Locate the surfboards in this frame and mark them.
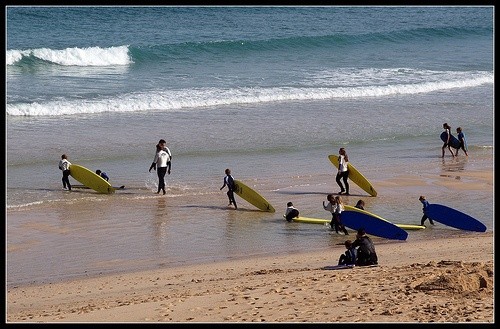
[340,204,408,241]
[68,183,126,190]
[328,155,378,197]
[284,214,425,230]
[423,203,486,233]
[232,180,275,212]
[439,130,462,149]
[68,164,115,194]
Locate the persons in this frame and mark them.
[59,155,72,190]
[336,148,350,195]
[353,200,364,210]
[149,139,172,195]
[286,202,299,223]
[351,228,375,265]
[455,127,468,156]
[338,240,359,267]
[220,169,237,210]
[441,123,455,157]
[323,194,350,236]
[96,170,109,181]
[419,196,434,226]
